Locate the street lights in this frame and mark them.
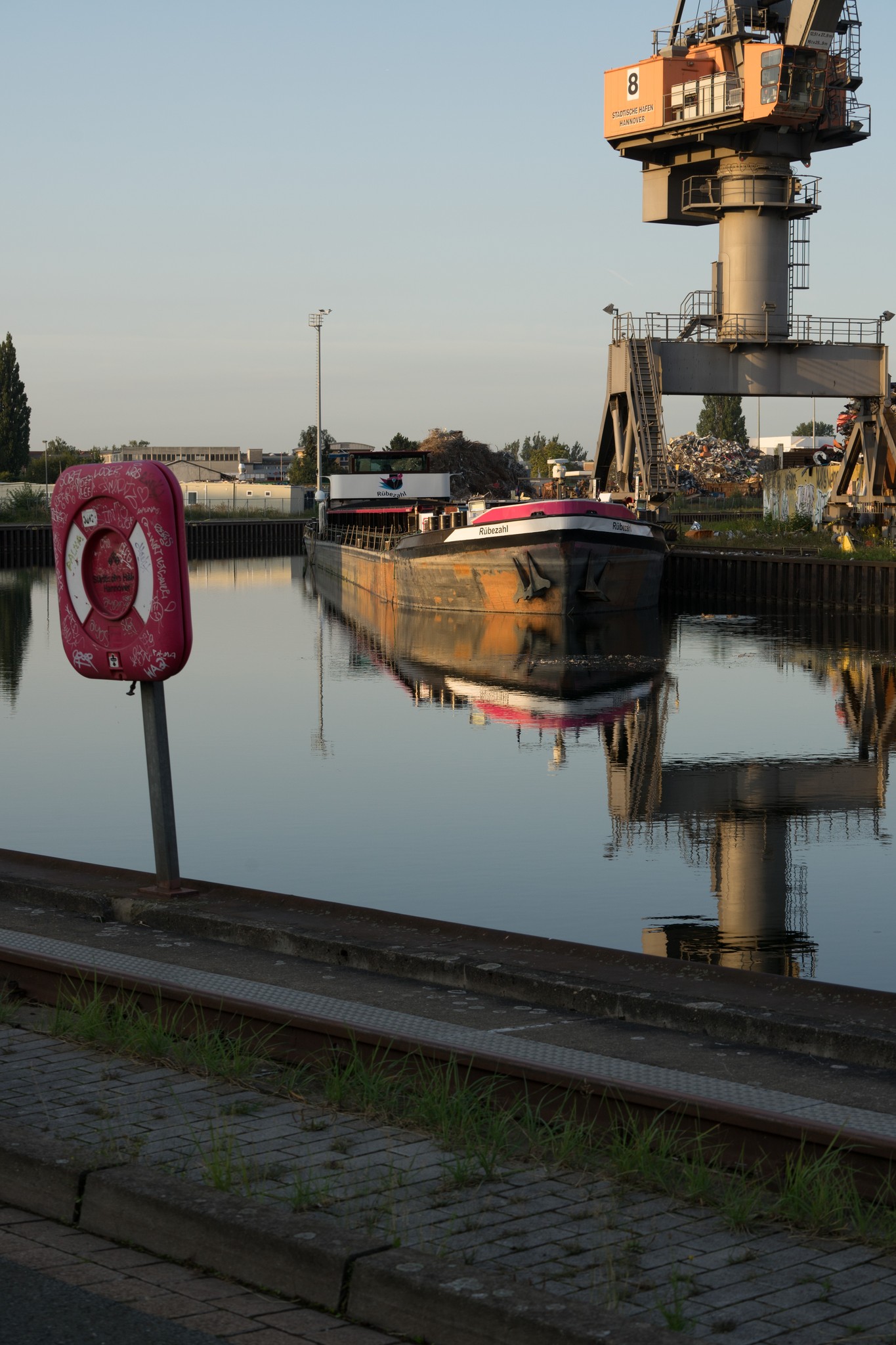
[42,441,48,509]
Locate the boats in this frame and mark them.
[303,451,675,689]
